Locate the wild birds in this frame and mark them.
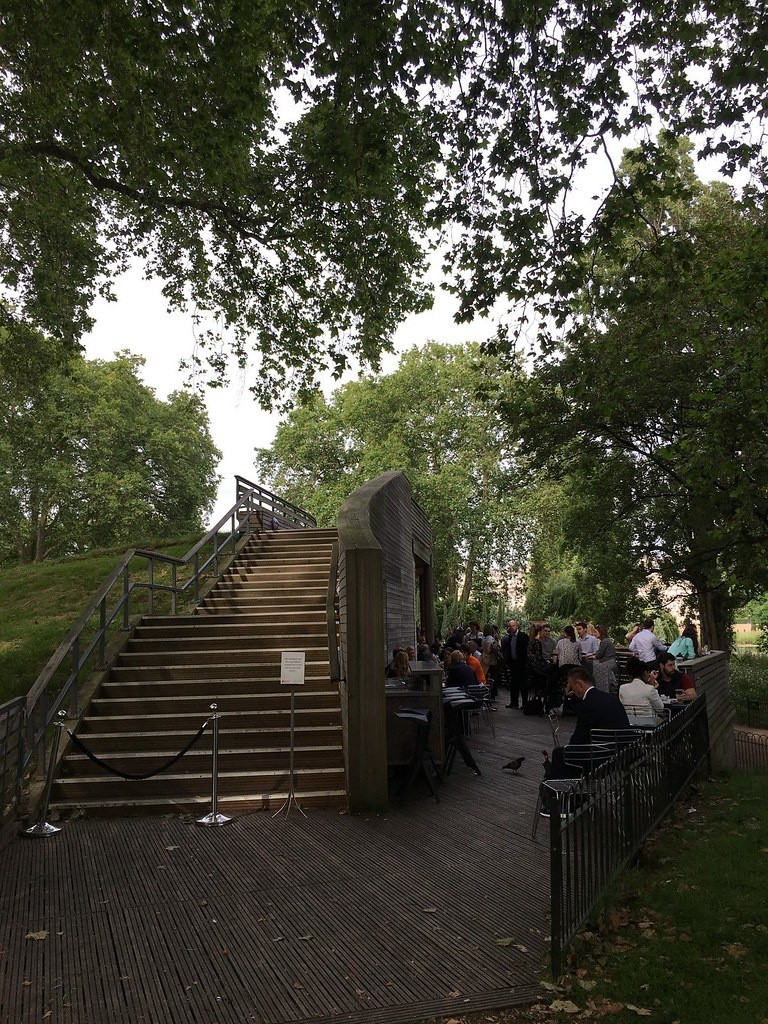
[503,757,525,773]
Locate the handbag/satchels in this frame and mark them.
[483,644,499,665]
[524,699,543,715]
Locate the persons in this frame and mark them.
[618,657,665,724]
[539,668,631,818]
[624,617,702,672]
[384,620,621,716]
[648,652,696,704]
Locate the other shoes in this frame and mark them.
[539,807,574,818]
[506,702,518,709]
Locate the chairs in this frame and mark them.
[532,703,685,839]
[446,672,497,741]
